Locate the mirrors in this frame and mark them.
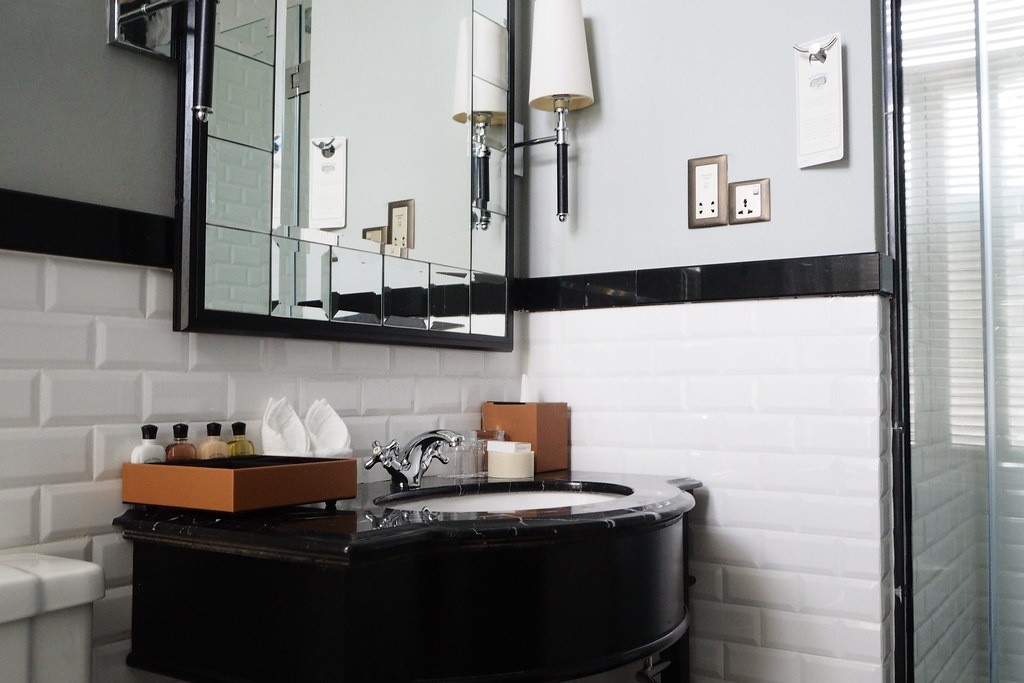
[169,0,519,356]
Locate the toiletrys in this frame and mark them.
[129,422,256,506]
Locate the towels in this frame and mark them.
[261,396,354,510]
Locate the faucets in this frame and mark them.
[390,429,464,489]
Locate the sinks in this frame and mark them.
[373,480,635,512]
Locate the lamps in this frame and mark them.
[456,11,512,231]
[514,1,596,225]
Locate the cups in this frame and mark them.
[438,430,477,478]
[466,428,506,477]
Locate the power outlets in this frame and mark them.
[385,199,416,259]
[727,174,773,227]
[362,225,388,277]
[685,153,732,231]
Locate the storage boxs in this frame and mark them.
[480,399,571,475]
